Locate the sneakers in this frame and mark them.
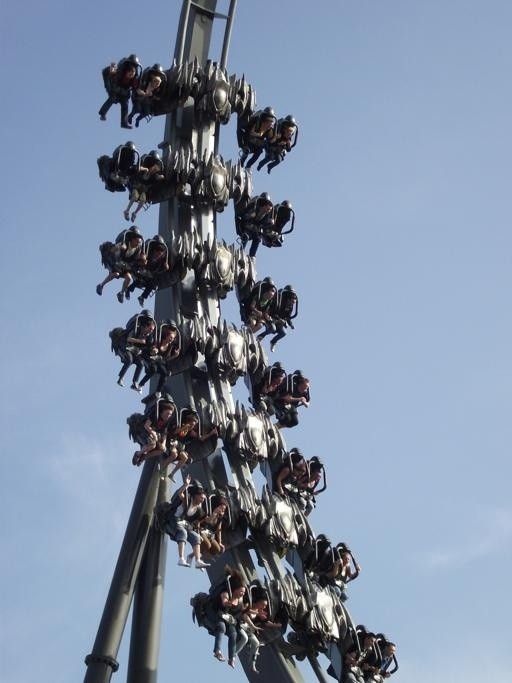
[96,283,144,307]
[138,386,143,395]
[155,392,161,401]
[241,160,272,176]
[251,256,256,264]
[256,335,263,345]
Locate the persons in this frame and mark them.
[97,58,398,681]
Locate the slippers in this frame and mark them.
[228,660,235,668]
[214,653,225,662]
[250,667,260,675]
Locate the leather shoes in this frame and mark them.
[117,380,125,388]
[130,383,141,391]
[178,555,211,573]
[135,118,139,127]
[124,211,130,220]
[132,451,176,484]
[128,115,132,124]
[131,213,137,222]
[270,341,275,352]
[100,115,106,120]
[121,124,132,129]
